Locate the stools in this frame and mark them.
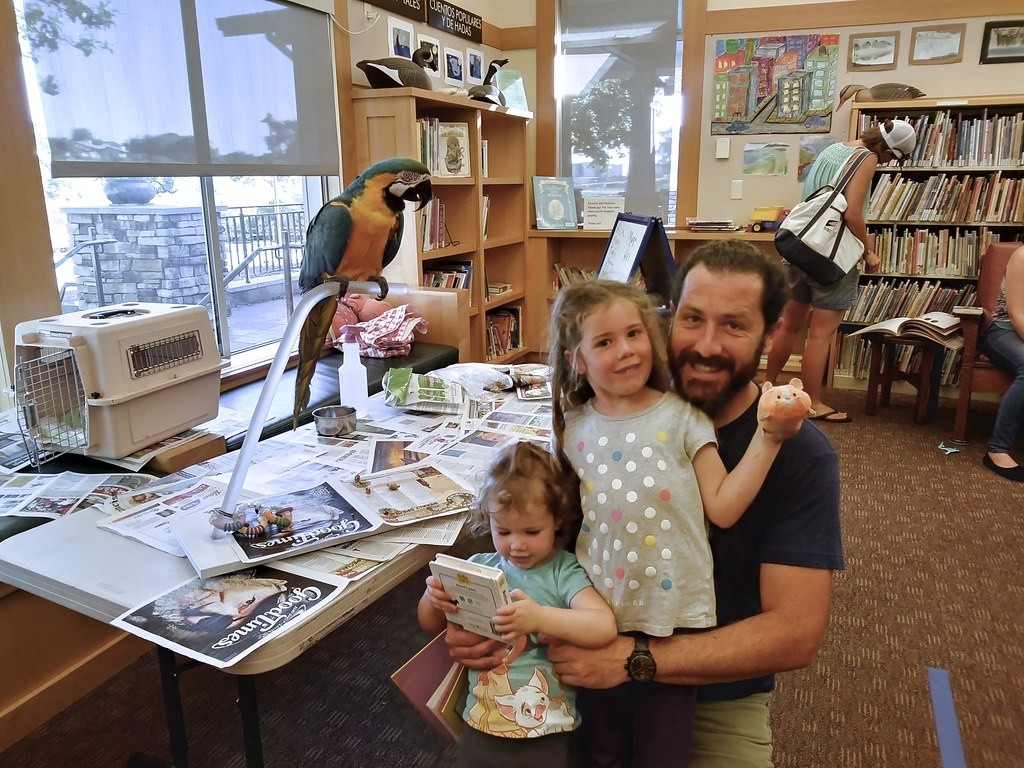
[861,331,944,425]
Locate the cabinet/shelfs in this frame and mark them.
[530,228,812,373]
[825,95,1024,381]
[350,86,533,364]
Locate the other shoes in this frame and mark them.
[982,450,1024,483]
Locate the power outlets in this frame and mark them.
[364,2,374,28]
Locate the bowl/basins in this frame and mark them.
[311,405,357,436]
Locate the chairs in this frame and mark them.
[951,243,1024,446]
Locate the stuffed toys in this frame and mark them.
[757,378,816,442]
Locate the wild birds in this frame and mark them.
[469,58,509,107]
[356,46,439,91]
[836,82,928,112]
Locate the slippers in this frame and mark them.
[808,407,854,424]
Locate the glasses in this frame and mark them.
[893,147,911,163]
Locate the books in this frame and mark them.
[858,110,1024,168]
[832,278,978,388]
[552,262,648,296]
[685,217,736,233]
[417,116,524,363]
[864,170,1024,223]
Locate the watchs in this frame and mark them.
[864,248,871,254]
[624,637,657,683]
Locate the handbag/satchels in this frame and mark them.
[775,147,878,287]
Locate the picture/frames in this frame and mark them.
[387,17,414,64]
[978,20,1024,63]
[907,24,969,65]
[846,31,901,72]
[444,46,465,87]
[438,121,471,179]
[416,35,441,80]
[466,48,485,86]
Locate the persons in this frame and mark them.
[856,224,1000,277]
[418,280,816,768]
[758,120,916,422]
[122,570,314,634]
[983,244,1024,482]
[443,238,846,768]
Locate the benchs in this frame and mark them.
[1,326,459,560]
[0,575,148,754]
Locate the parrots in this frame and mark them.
[291,157,434,432]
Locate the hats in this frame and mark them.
[879,119,916,167]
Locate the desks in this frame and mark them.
[0,362,557,768]
